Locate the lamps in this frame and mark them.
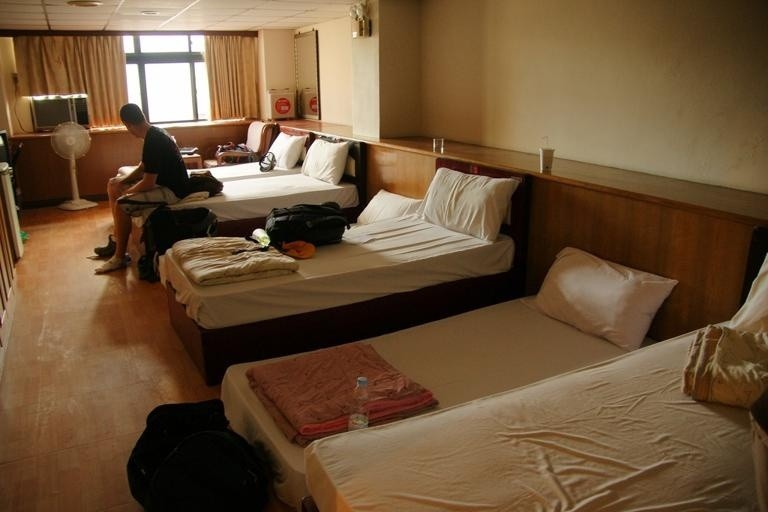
[347,0,372,19]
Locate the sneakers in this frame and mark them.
[93,234,118,258]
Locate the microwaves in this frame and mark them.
[29,92,91,132]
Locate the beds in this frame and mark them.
[165,211,516,385]
[305,319,768,512]
[116,135,366,254]
[219,295,661,512]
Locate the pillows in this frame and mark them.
[264,131,308,171]
[521,246,677,353]
[299,138,349,187]
[357,189,423,223]
[728,251,768,335]
[413,166,521,246]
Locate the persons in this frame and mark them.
[94,103,189,274]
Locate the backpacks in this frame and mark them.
[262,200,353,251]
[190,171,223,198]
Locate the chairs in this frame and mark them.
[204,121,280,168]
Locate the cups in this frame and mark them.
[539,145,556,172]
[431,138,447,152]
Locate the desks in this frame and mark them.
[181,153,203,169]
[9,120,254,210]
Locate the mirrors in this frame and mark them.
[294,30,320,120]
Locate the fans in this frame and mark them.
[49,121,98,210]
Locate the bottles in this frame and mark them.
[346,376,370,431]
[251,228,271,247]
